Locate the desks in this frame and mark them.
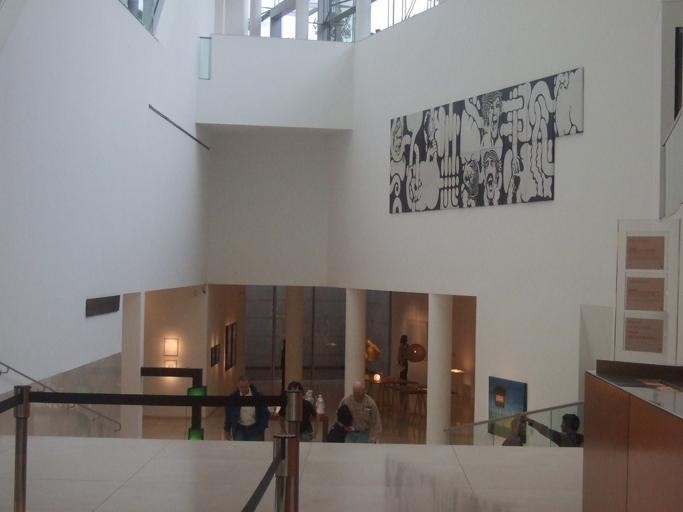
[365,373,457,428]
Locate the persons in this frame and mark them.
[396,333,411,385]
[278,337,285,387]
[222,374,270,441]
[515,410,584,446]
[335,379,383,444]
[501,417,525,445]
[277,380,319,442]
[302,388,325,414]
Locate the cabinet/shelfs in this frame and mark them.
[581,368,683,512]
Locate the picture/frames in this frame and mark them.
[210,321,238,373]
[163,360,177,369]
[162,336,178,357]
